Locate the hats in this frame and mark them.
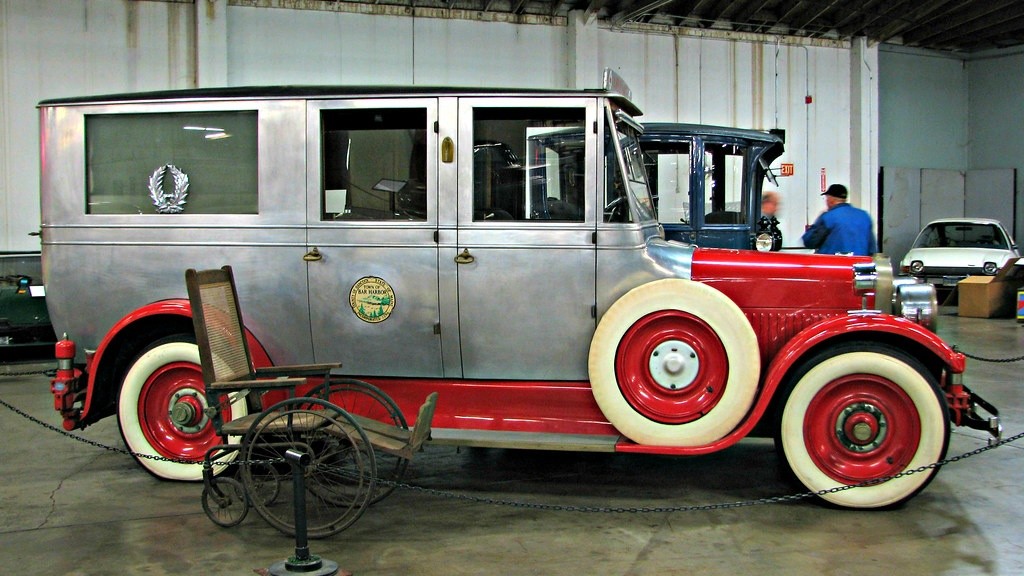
[821,184,848,199]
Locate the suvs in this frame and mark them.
[35,67,1002,509]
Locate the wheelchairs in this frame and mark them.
[185,266,437,540]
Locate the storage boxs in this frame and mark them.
[957,258,1021,319]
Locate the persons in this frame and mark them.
[802,184,876,256]
[761,191,779,228]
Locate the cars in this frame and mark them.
[0,250,60,361]
[899,218,1024,292]
